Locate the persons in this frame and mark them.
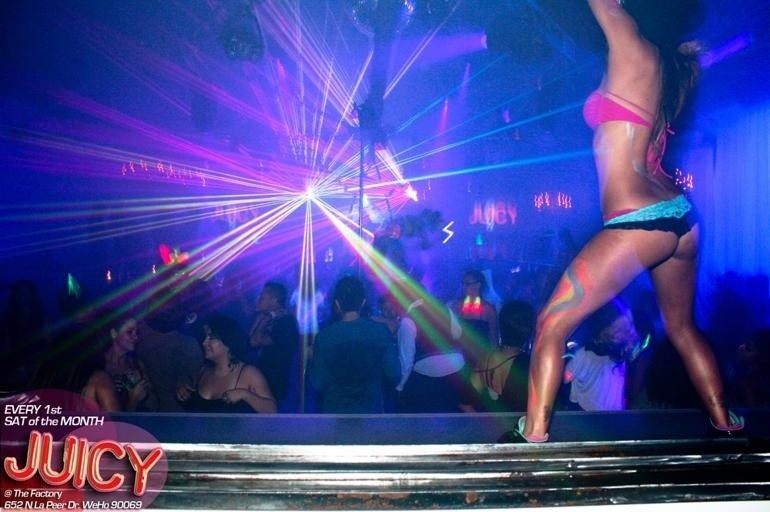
[371,208,447,280]
[2,265,769,412]
[493,1,750,443]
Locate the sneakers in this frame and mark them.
[708,410,745,437]
[499,416,550,442]
